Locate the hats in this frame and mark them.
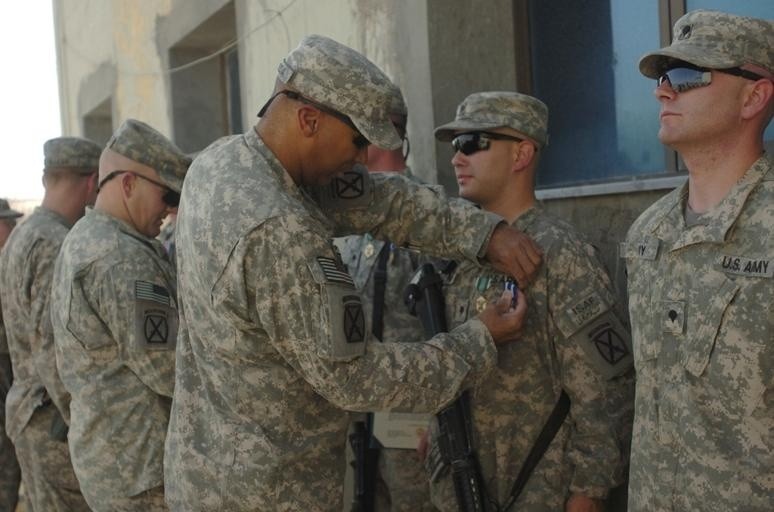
[434,92,548,143]
[44,136,98,176]
[638,8,773,80]
[277,38,404,150]
[98,120,191,194]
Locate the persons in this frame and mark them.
[433,90,634,512]
[160,34,544,510]
[1,118,194,512]
[618,9,774,511]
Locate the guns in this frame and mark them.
[403,265,483,511]
[349,419,377,511]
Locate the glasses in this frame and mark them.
[99,171,177,210]
[452,131,520,154]
[657,63,760,95]
[280,90,370,149]
